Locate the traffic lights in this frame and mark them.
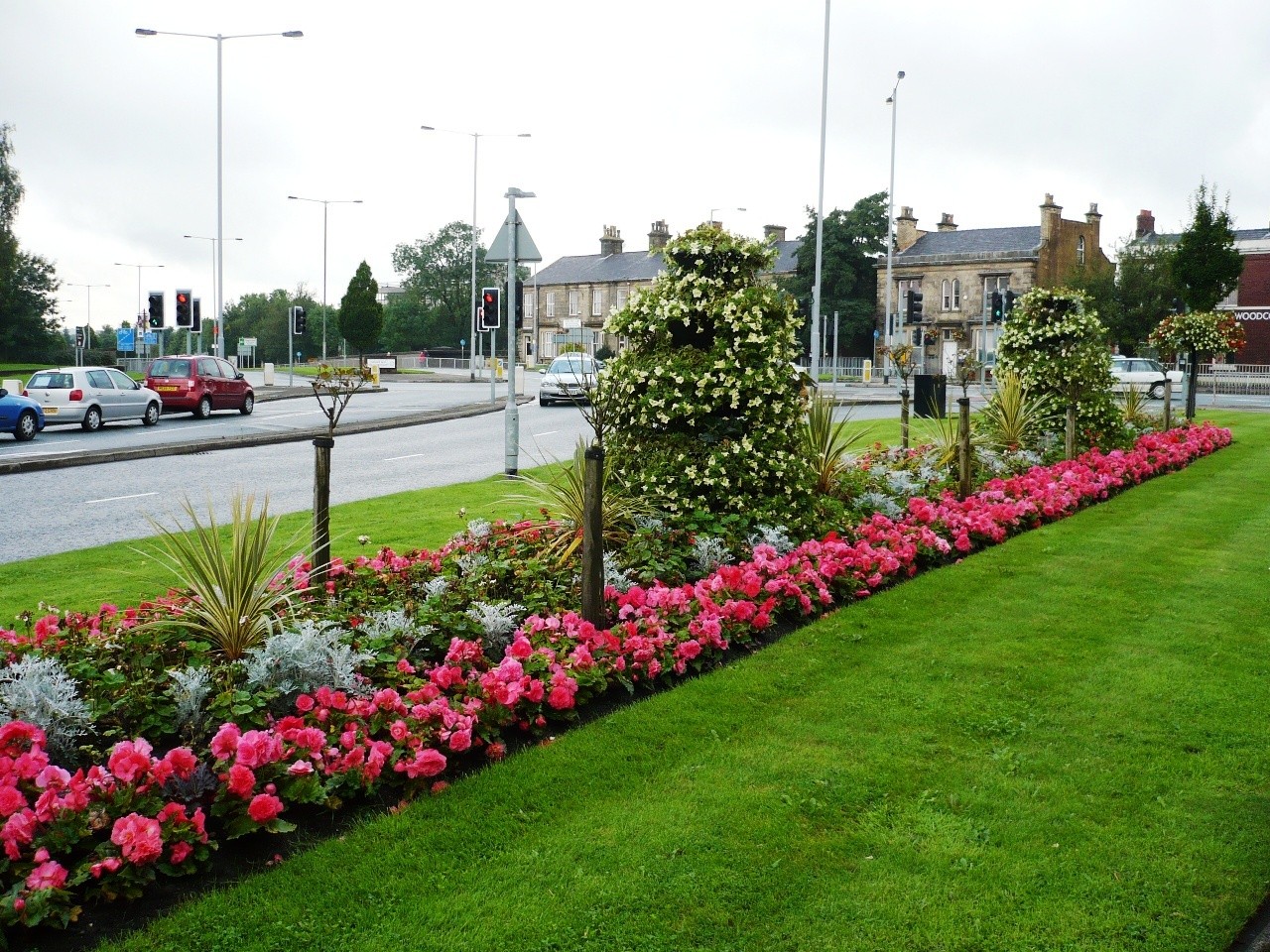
[924,334,930,345]
[477,307,490,333]
[481,287,501,329]
[912,327,921,346]
[294,305,306,335]
[906,290,923,324]
[176,289,194,328]
[991,292,1003,323]
[148,292,165,330]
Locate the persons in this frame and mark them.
[420,351,425,368]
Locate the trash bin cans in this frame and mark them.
[914,374,946,418]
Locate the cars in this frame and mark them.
[1101,354,1185,400]
[0,386,46,441]
[555,351,606,369]
[537,356,603,407]
[21,365,163,432]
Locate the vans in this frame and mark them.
[142,353,256,420]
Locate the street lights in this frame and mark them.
[420,124,531,382]
[183,234,244,356]
[882,70,906,384]
[133,27,304,362]
[67,283,111,349]
[287,195,363,378]
[114,263,166,358]
[710,207,747,228]
